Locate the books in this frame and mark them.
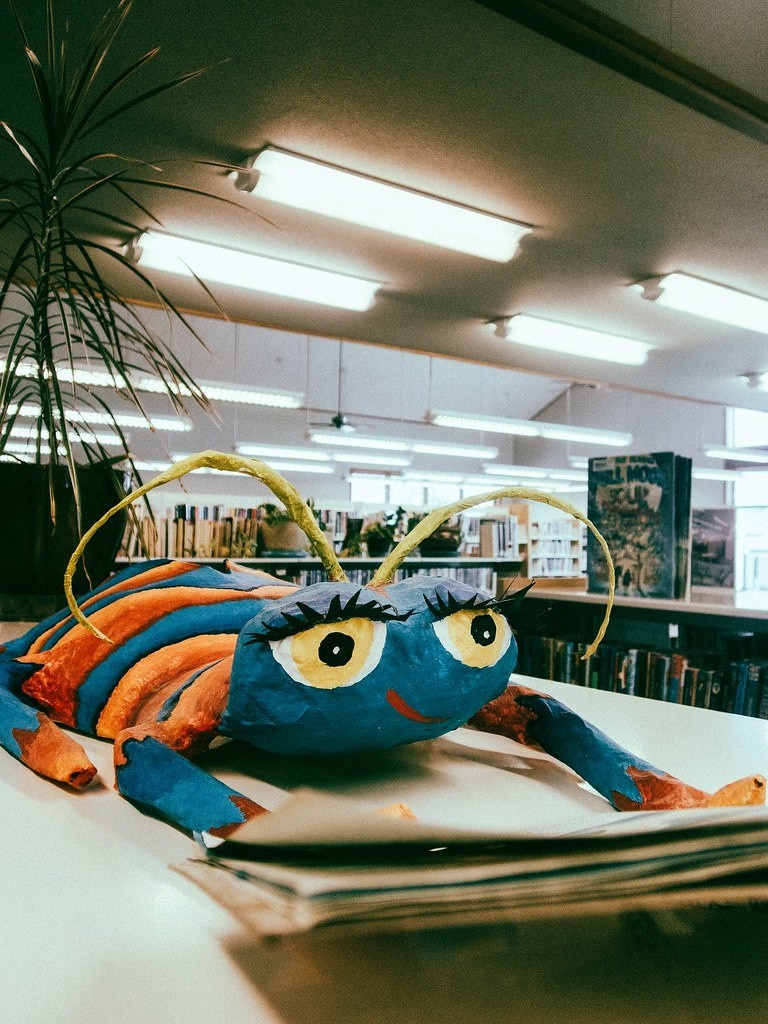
[519,635,768,721]
[119,505,518,596]
[169,788,768,948]
[532,520,575,573]
[585,451,692,599]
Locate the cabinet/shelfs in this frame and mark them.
[107,498,768,720]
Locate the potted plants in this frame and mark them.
[0,0,280,623]
